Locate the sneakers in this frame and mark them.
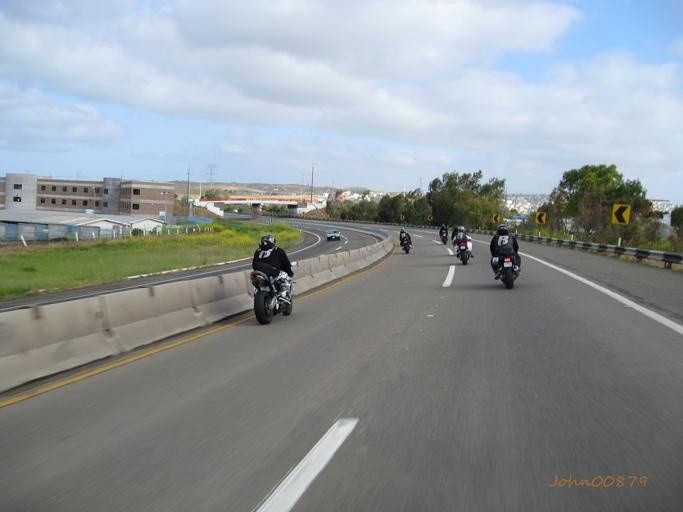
[495,272,501,280]
[277,295,291,305]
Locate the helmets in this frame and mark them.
[497,224,508,235]
[261,235,276,249]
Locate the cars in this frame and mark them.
[326,229,341,241]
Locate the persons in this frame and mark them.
[489,224,521,280]
[252,234,294,305]
[452,224,473,257]
[399,227,412,248]
[439,224,448,238]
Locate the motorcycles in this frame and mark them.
[488,247,521,289]
[440,229,448,245]
[452,239,474,265]
[248,260,300,326]
[400,234,413,255]
